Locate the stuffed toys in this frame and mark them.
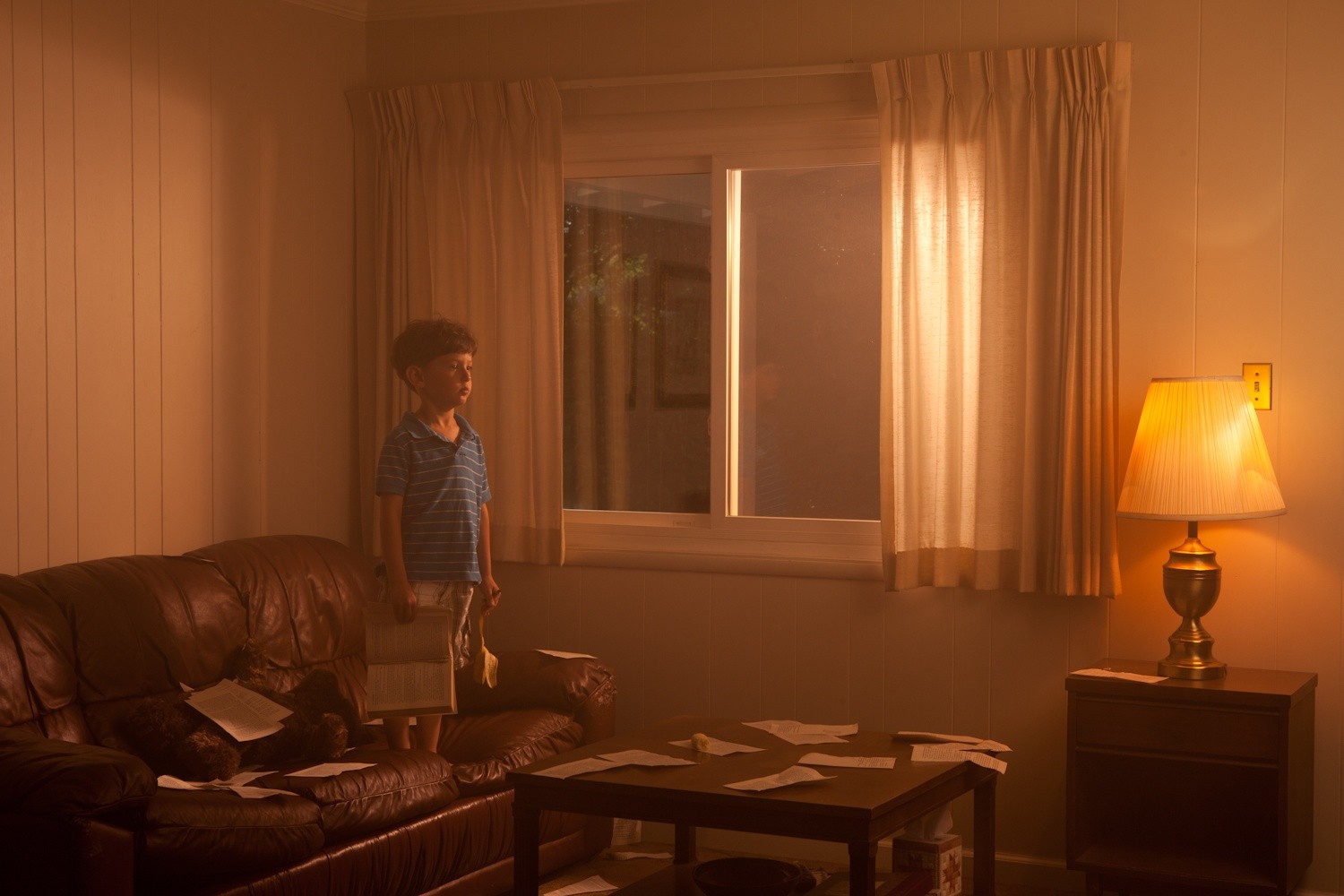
[127,641,370,782]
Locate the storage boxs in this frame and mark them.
[892,833,963,896]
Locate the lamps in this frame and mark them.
[1109,379,1289,681]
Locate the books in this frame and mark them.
[362,607,458,720]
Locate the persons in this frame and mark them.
[376,321,501,767]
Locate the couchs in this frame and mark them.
[1,535,619,896]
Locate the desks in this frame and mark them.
[1056,669,1318,896]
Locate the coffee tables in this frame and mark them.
[508,729,1003,896]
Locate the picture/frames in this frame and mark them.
[655,266,713,406]
